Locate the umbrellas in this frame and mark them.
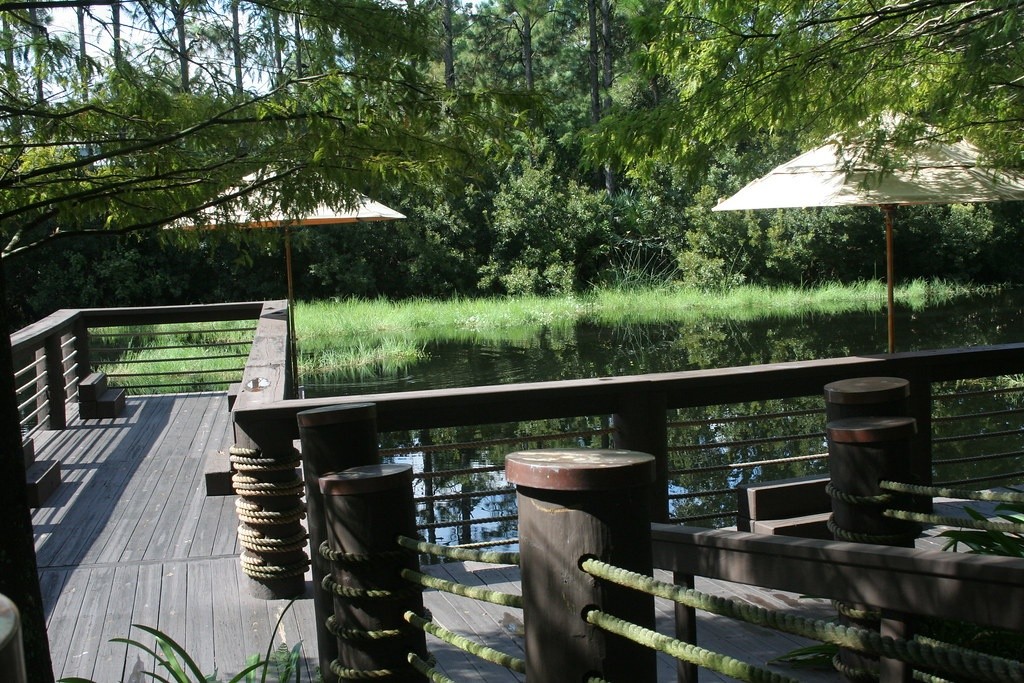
[710,107,1024,354]
[162,154,406,393]
